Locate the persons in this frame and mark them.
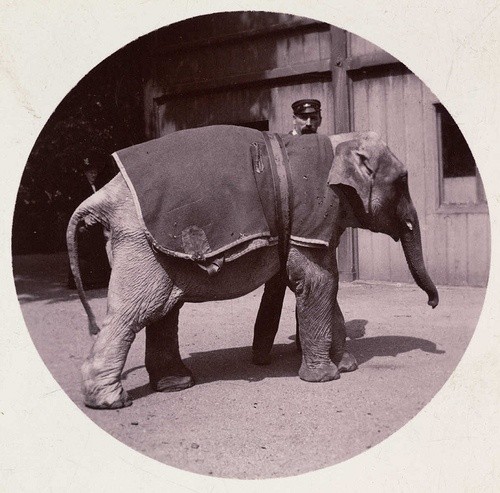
[251,98,323,365]
[72,152,104,259]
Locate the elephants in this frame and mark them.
[66,125,439,409]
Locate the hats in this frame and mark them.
[291,99,321,115]
